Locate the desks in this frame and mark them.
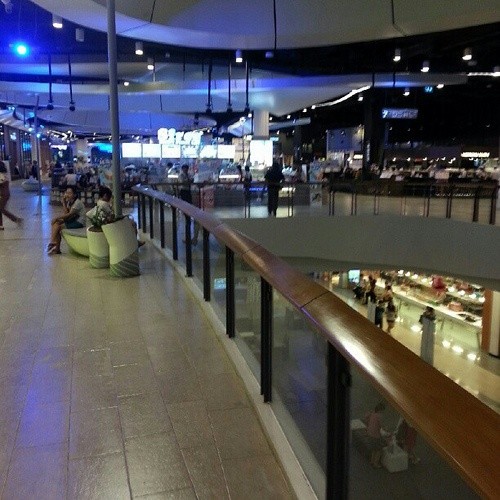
[381,445,408,473]
[365,278,482,350]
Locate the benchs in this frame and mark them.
[48,188,131,206]
[60,229,88,257]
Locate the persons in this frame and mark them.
[0,161,24,230]
[240,159,500,217]
[367,403,424,469]
[31,156,193,255]
[353,274,446,335]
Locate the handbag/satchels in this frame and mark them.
[86,206,98,218]
[64,214,79,223]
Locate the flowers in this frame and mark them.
[86,200,114,227]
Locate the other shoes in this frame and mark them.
[0,228,4,230]
[54,246,61,254]
[138,241,145,247]
[47,244,57,253]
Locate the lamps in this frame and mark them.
[463,47,472,60]
[422,60,430,73]
[53,14,62,28]
[395,48,402,61]
[135,41,143,55]
[236,50,242,63]
[147,57,154,69]
[76,28,84,42]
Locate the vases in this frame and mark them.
[101,216,140,277]
[87,225,109,269]
